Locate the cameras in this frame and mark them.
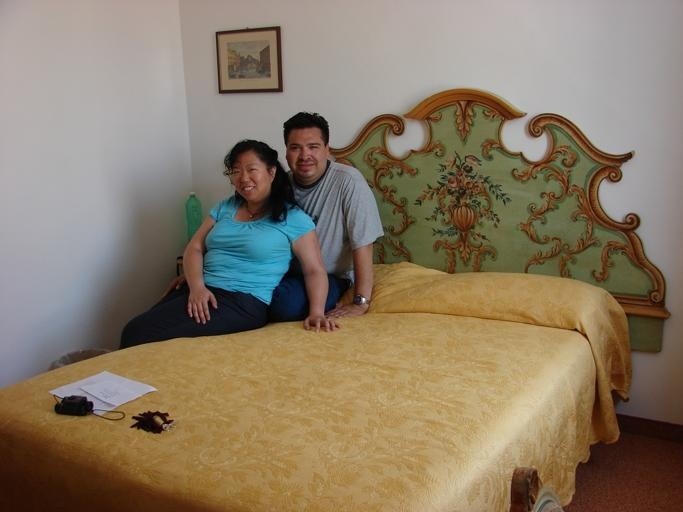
[55,394,94,416]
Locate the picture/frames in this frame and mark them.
[215,27,283,93]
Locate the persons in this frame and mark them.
[115,138,343,349]
[157,111,385,322]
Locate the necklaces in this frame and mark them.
[246,208,259,222]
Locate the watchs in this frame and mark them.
[352,294,370,305]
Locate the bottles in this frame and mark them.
[186,191,203,241]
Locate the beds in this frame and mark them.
[0,89,670,512]
